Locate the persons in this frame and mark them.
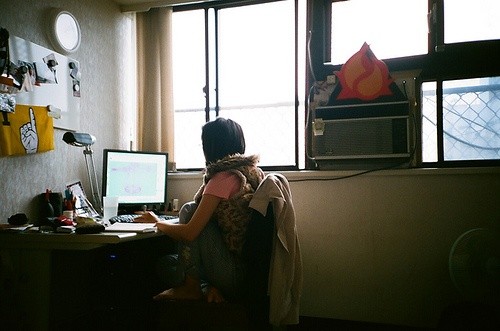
[132,117,263,304]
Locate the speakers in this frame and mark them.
[311,100,410,160]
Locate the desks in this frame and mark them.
[0,211,179,331]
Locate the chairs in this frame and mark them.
[155,173,301,331]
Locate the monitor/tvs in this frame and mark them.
[101,149,168,205]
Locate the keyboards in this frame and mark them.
[110,212,178,224]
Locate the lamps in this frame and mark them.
[62,131,103,216]
